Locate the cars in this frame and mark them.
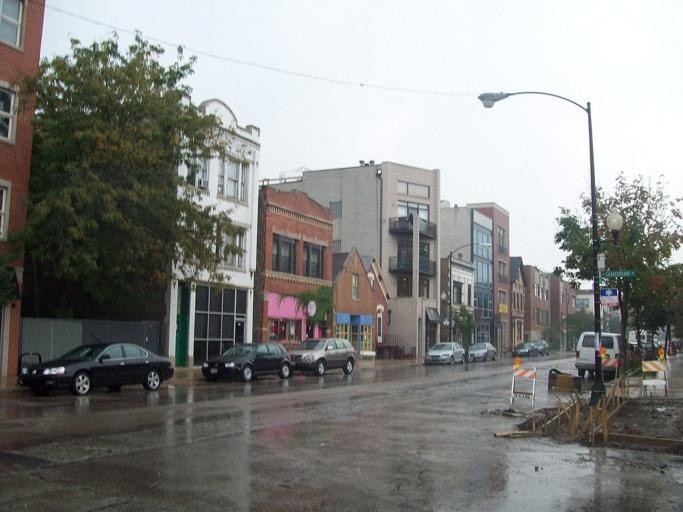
[510,342,536,357]
[468,341,496,361]
[200,343,291,384]
[626,329,680,359]
[533,339,549,356]
[17,342,174,396]
[426,341,465,365]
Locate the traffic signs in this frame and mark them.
[602,269,636,277]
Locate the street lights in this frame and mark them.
[447,242,492,342]
[479,88,624,409]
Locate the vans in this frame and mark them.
[574,331,635,378]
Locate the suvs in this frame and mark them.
[289,337,356,376]
